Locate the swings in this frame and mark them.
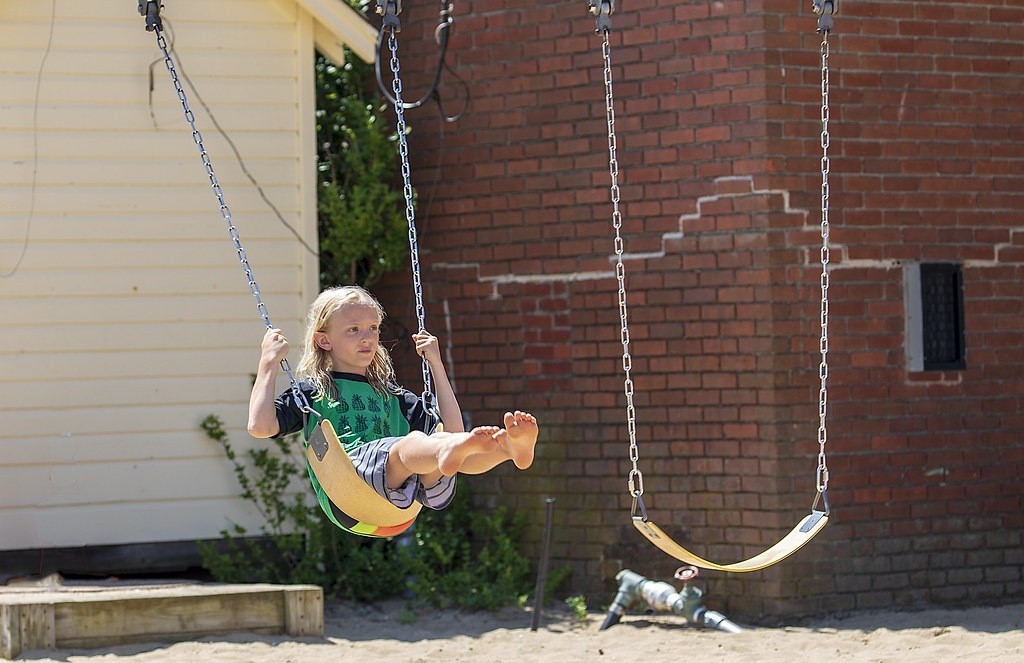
[585,1,832,572]
[136,0,448,541]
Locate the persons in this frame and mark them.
[247,286,539,540]
